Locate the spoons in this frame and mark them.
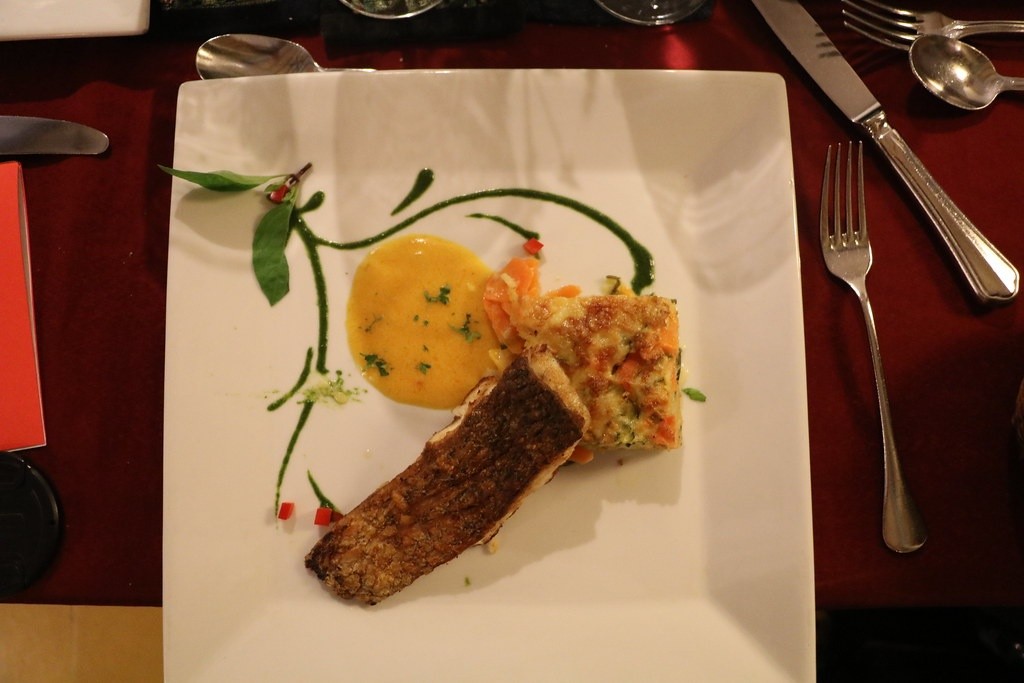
[195,35,376,80]
[910,35,1024,110]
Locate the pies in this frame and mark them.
[506,281,682,453]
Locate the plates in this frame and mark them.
[161,68,816,683]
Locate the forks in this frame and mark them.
[820,142,924,552]
[840,0,1024,50]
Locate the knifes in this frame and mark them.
[752,0,1016,304]
[0,115,108,154]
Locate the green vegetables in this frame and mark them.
[161,166,312,302]
